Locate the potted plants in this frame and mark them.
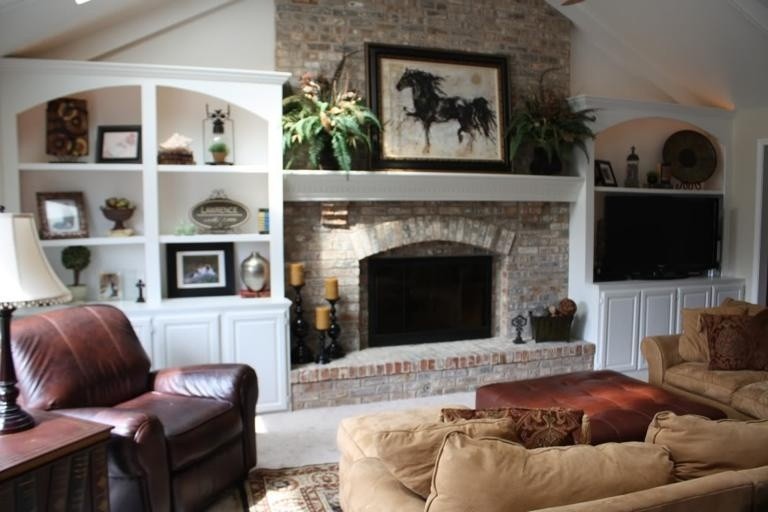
[283,44,383,182]
[209,142,231,163]
[505,64,597,176]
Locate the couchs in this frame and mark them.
[335,404,767,511]
[640,299,768,419]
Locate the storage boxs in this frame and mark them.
[529,309,572,343]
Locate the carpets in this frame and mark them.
[246,459,339,512]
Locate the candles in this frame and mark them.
[315,306,332,330]
[324,277,340,299]
[289,262,307,286]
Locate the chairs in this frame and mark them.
[7,301,260,512]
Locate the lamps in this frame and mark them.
[0,208,74,432]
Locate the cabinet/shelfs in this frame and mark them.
[0,406,111,511]
[677,281,742,337]
[151,297,291,417]
[598,284,678,376]
[0,54,293,300]
[587,95,739,282]
[94,302,152,372]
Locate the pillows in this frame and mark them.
[679,301,750,363]
[701,310,768,372]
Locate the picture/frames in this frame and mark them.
[362,38,515,174]
[165,241,237,299]
[595,159,618,187]
[94,123,142,165]
[34,190,90,240]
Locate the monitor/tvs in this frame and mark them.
[367,254,496,349]
[597,192,725,283]
